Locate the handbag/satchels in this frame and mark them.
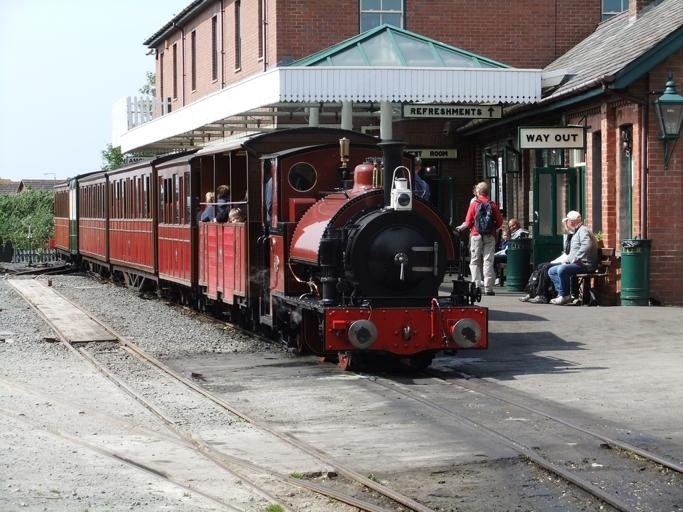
[529,271,540,288]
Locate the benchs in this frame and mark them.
[572,247,617,304]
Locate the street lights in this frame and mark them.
[42,172,56,186]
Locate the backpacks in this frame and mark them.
[475,199,496,234]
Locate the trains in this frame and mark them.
[51,120,491,384]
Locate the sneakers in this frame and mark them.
[485,291,495,296]
[520,294,571,304]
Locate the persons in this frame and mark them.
[518,214,572,303]
[546,209,597,303]
[199,161,272,224]
[415,155,429,200]
[493,227,510,253]
[450,180,502,297]
[491,218,524,287]
[468,180,501,287]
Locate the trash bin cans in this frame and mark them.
[620,239,652,306]
[506,238,532,292]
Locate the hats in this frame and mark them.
[567,210,581,220]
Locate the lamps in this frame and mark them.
[647,67,683,172]
